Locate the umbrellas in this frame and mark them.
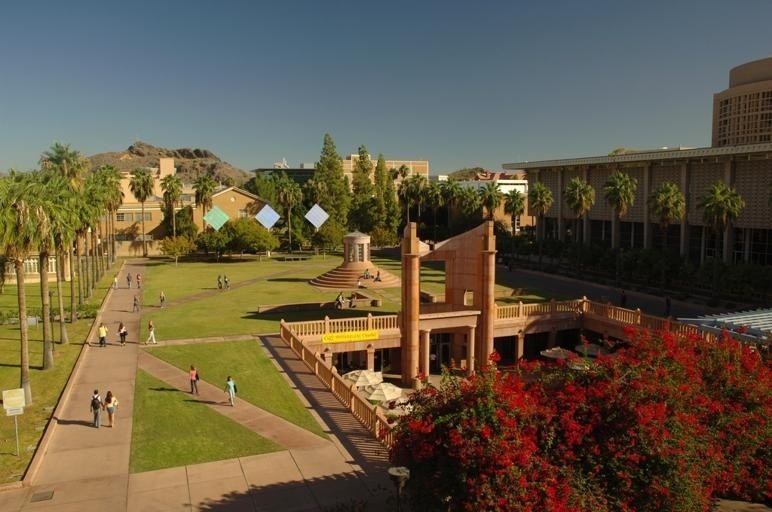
[342,368,384,388]
[358,382,403,402]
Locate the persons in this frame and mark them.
[144,320,158,346]
[90,389,105,428]
[349,291,356,309]
[335,291,345,310]
[336,355,391,375]
[137,273,142,289]
[117,322,128,347]
[364,268,371,279]
[113,274,119,290]
[189,364,199,394]
[224,274,231,291]
[103,391,120,428]
[133,294,142,311]
[223,375,237,407]
[126,272,133,289]
[159,291,167,310]
[374,271,382,282]
[216,275,223,291]
[430,351,440,371]
[97,322,109,347]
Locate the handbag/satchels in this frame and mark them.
[234,385,237,393]
[196,374,199,380]
[124,332,128,335]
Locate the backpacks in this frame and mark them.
[92,395,100,410]
